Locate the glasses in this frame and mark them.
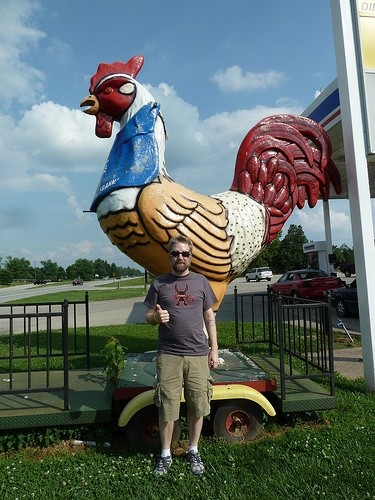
[171,251,190,257]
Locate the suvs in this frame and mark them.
[245,266,272,282]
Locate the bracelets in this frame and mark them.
[154,312,160,323]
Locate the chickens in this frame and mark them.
[79,56,342,368]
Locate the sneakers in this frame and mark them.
[153,456,173,477]
[188,449,205,474]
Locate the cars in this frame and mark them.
[331,278,360,318]
[267,268,345,305]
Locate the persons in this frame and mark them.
[146,235,218,475]
